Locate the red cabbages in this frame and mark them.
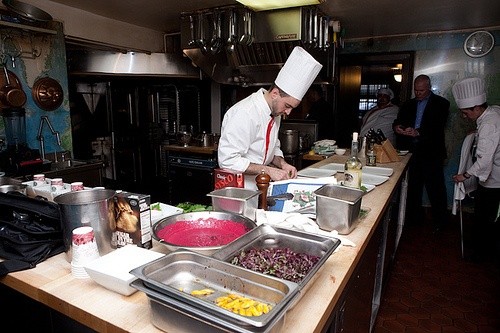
[232,248,320,283]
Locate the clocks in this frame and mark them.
[464,30,494,59]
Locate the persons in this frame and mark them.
[217,45,323,192]
[451,77,500,266]
[358,87,400,145]
[393,74,451,228]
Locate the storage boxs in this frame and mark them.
[312,184,367,234]
[215,168,243,190]
[150,201,182,228]
[111,193,150,248]
[207,187,263,216]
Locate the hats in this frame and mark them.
[275,46,323,102]
[376,87,394,100]
[452,78,487,108]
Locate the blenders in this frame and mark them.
[0,108,42,175]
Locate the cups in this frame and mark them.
[70,226,102,279]
[70,182,84,191]
[33,174,45,181]
[51,178,63,187]
[92,187,105,190]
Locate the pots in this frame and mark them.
[2,0,53,22]
[178,133,192,146]
[197,134,213,147]
[0,63,26,107]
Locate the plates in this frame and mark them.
[296,162,394,193]
[397,151,409,156]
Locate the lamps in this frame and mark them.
[394,69,402,82]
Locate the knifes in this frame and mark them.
[365,128,386,145]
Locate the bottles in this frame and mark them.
[344,140,362,190]
[358,137,376,166]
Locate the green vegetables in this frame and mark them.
[361,186,368,192]
[359,209,367,217]
[177,202,213,212]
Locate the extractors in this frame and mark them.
[180,5,342,88]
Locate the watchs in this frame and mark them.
[463,172,469,178]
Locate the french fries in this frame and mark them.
[192,289,269,316]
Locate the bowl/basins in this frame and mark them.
[150,210,258,257]
[334,149,346,155]
[83,243,167,296]
[0,184,28,195]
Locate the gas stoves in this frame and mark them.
[168,153,217,169]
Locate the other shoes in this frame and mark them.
[431,223,446,232]
[463,255,496,264]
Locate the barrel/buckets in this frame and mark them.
[53,189,118,264]
[279,128,298,158]
